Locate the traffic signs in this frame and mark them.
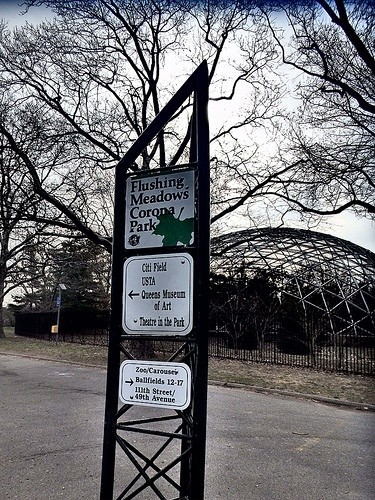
[117,360,191,410]
[120,253,194,337]
